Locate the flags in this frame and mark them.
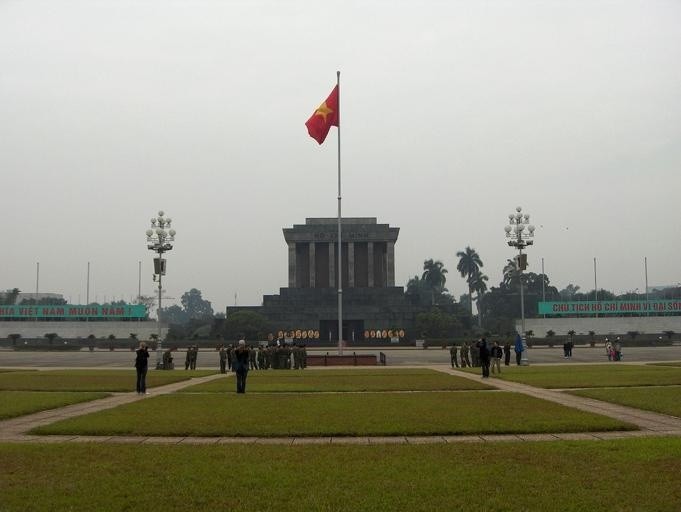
[305,85,339,146]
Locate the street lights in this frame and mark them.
[504,206,535,367]
[146,211,176,362]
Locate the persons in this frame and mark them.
[135,342,151,393]
[568,337,572,356]
[563,341,568,359]
[450,338,510,379]
[604,335,623,361]
[161,342,309,371]
[232,339,252,394]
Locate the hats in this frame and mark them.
[239,340,245,345]
